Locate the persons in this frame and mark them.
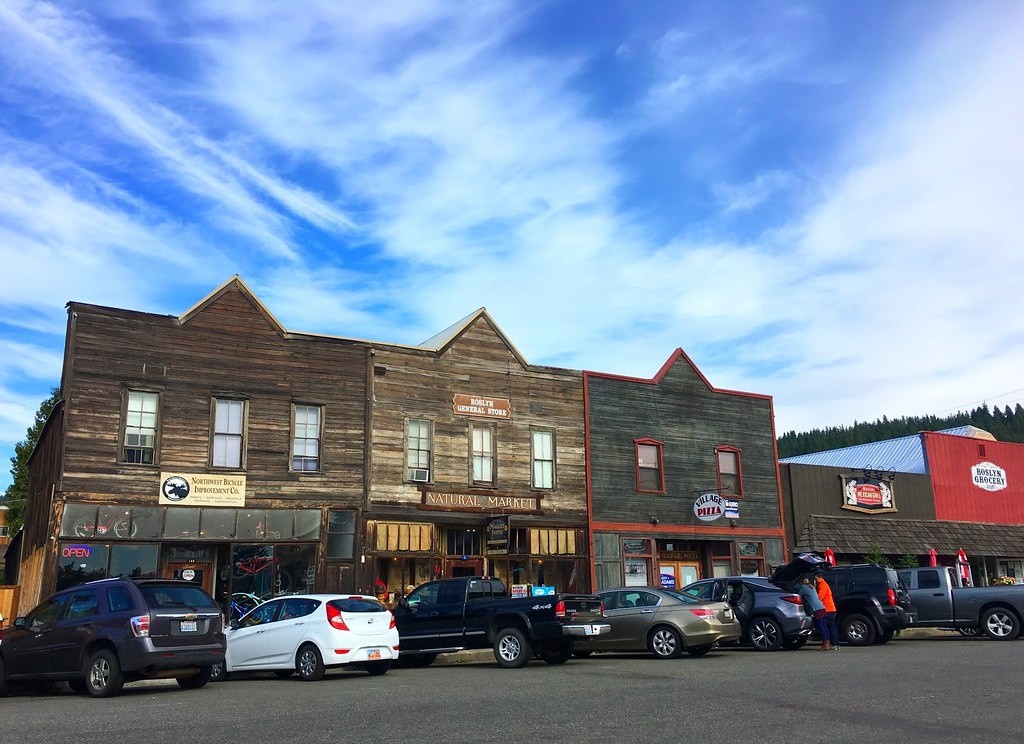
[374,579,401,610]
[813,571,840,651]
[797,576,831,651]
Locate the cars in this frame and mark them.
[677,552,831,652]
[211,594,400,682]
[0,576,228,697]
[570,584,742,661]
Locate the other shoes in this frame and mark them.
[830,644,840,651]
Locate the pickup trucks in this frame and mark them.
[890,567,1024,642]
[390,575,614,669]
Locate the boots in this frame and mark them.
[818,640,830,651]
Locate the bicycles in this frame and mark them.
[230,547,292,597]
[222,593,264,625]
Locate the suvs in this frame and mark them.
[810,562,920,648]
